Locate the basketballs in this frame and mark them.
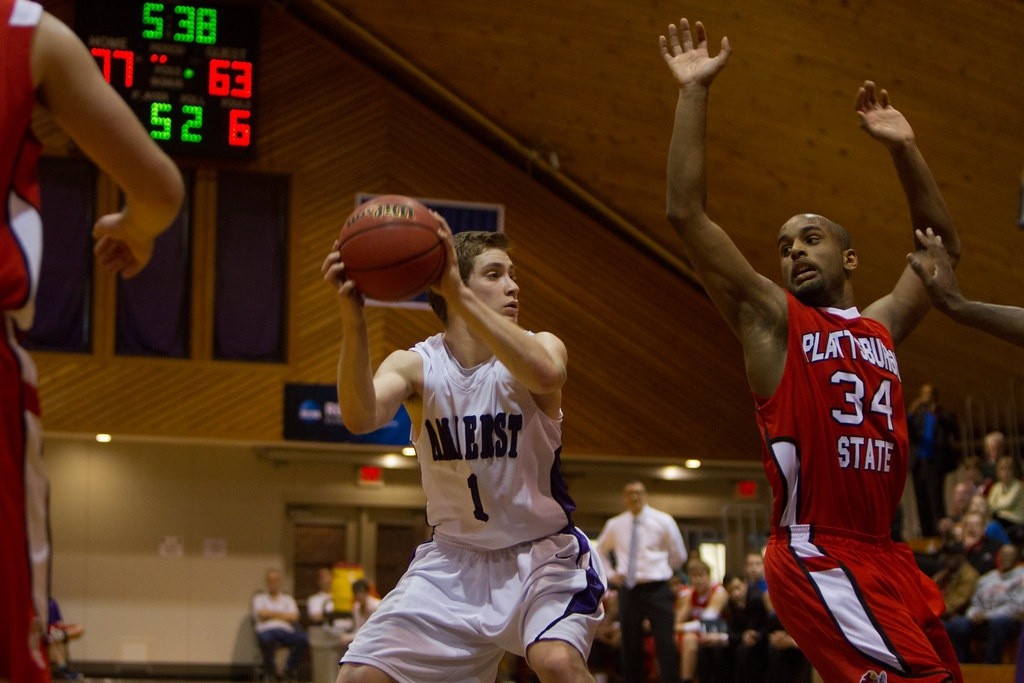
[337,192,449,304]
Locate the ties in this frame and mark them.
[623,517,640,591]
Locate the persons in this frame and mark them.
[44,595,85,683]
[319,228,608,683]
[0,1,187,683]
[594,478,690,683]
[655,15,966,683]
[904,373,1024,665]
[248,561,384,683]
[901,224,1024,344]
[597,540,815,683]
